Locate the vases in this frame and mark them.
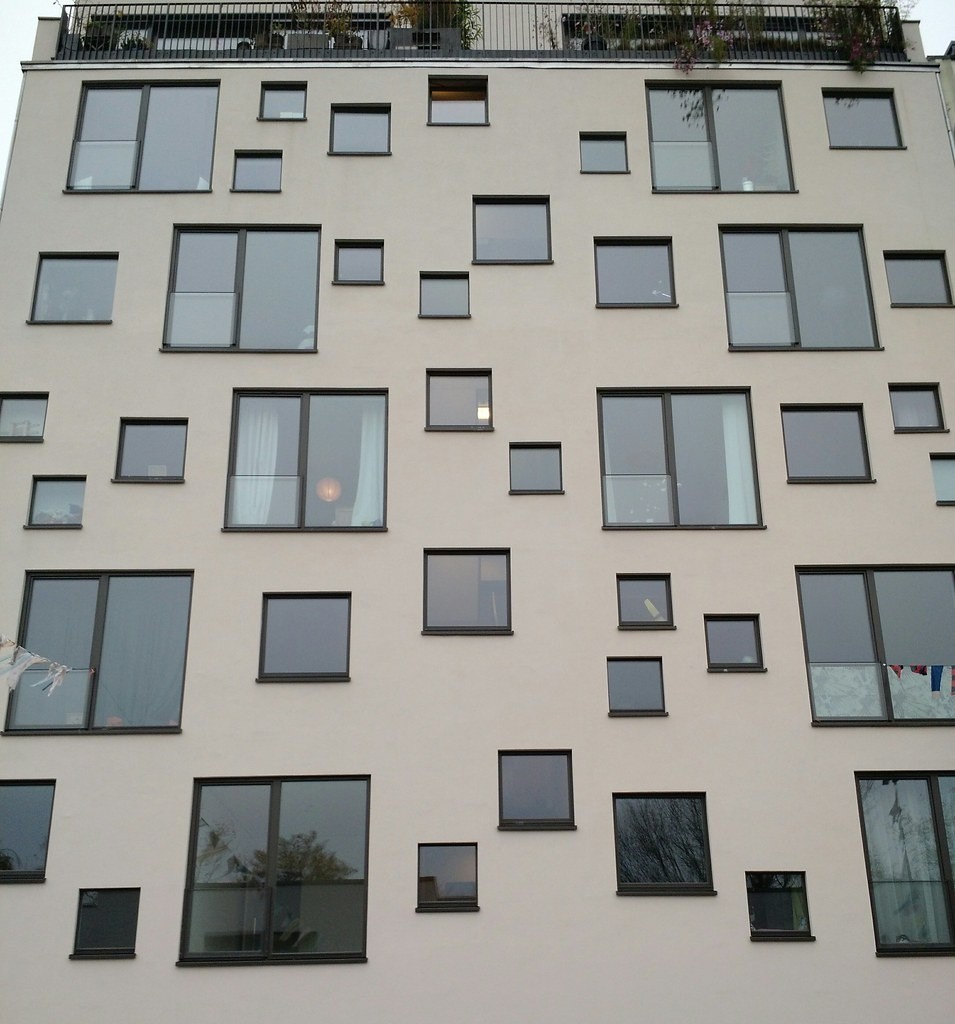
[581,36,608,50]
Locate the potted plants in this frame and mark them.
[248,0,483,50]
[82,10,157,51]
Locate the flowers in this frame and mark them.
[560,3,619,37]
